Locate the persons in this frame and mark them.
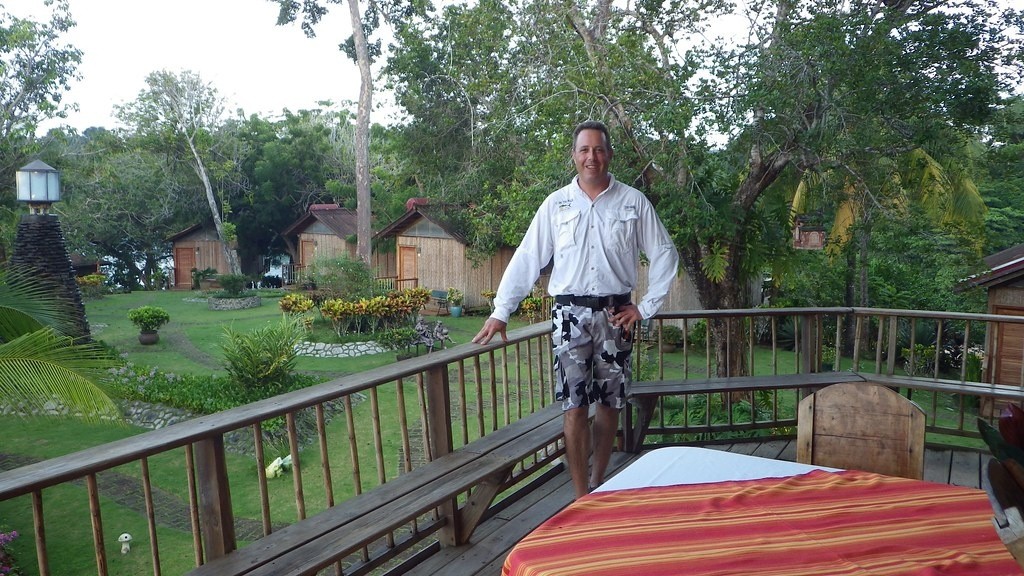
[472,121,679,501]
[415,317,435,354]
[433,320,456,350]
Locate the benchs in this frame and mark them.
[424,289,450,316]
[182,371,1024,576]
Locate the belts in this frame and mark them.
[555,292,631,309]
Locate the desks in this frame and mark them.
[501,447,1024,576]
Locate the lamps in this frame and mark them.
[14,159,62,215]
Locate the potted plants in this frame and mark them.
[446,287,464,318]
[304,276,316,291]
[379,327,421,361]
[821,345,836,372]
[652,325,682,353]
[127,306,170,345]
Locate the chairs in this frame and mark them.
[981,458,1024,571]
[796,381,926,481]
[634,318,651,345]
